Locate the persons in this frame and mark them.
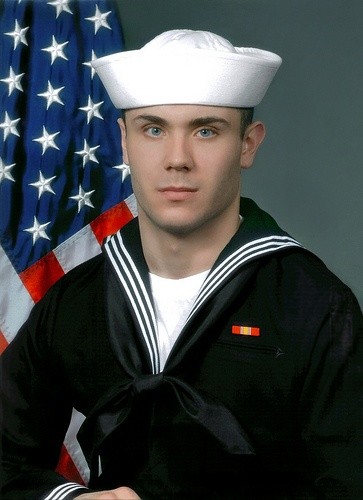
[0,29,362,500]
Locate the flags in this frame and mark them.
[0,0,140,490]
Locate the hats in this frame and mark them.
[90,29,282,110]
